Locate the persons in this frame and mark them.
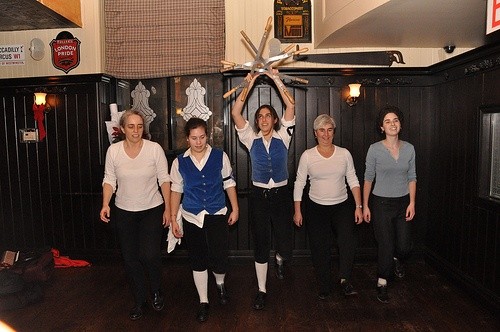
[169,118,239,322]
[100,111,170,320]
[293,114,363,299]
[232,69,296,311]
[362,108,417,303]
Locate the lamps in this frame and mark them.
[346,83,362,107]
[34,89,55,113]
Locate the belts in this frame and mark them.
[251,186,288,198]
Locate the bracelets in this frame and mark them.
[356,205,362,208]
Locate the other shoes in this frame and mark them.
[319,283,330,299]
[216,284,225,298]
[198,302,209,321]
[393,258,406,280]
[376,285,390,303]
[275,263,285,280]
[254,291,266,310]
[340,281,357,295]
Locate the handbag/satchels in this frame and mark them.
[13,252,55,282]
[0,270,31,312]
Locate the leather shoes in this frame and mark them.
[129,303,144,321]
[152,289,164,310]
